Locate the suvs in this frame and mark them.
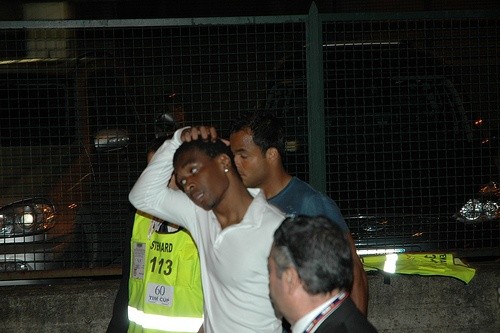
[239,39,499,268]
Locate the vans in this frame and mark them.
[0,53,184,280]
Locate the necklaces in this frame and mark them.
[302,290,348,333]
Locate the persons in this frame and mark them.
[106,138,205,333]
[267,215,379,333]
[128,126,289,333]
[230,111,369,319]
